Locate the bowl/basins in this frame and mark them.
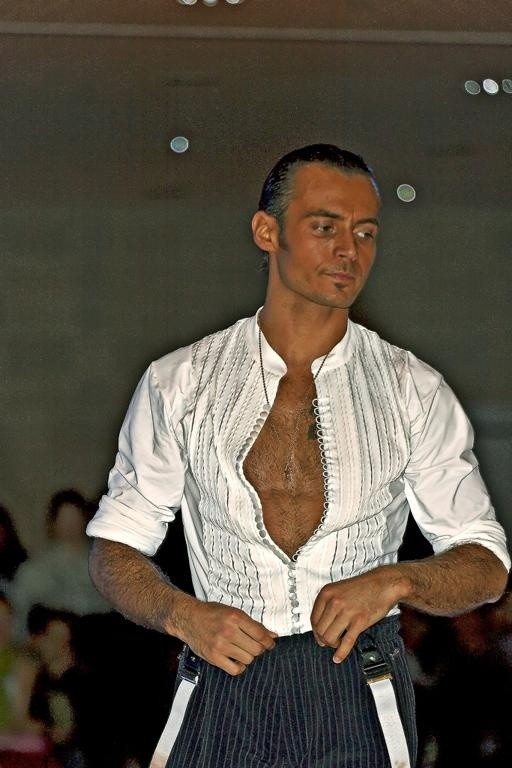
[257,306,335,489]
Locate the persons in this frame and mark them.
[87,145,510,767]
[398,510,511,767]
[2,488,196,766]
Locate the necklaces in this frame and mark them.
[257,306,335,489]
